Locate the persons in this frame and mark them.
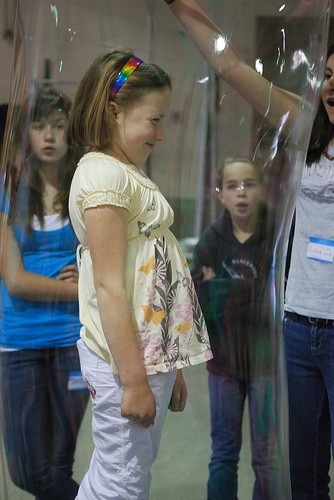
[187,155,291,500]
[52,51,213,500]
[166,0,334,500]
[0,86,88,500]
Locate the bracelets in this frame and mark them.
[163,0,175,4]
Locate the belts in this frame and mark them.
[284,310,334,328]
